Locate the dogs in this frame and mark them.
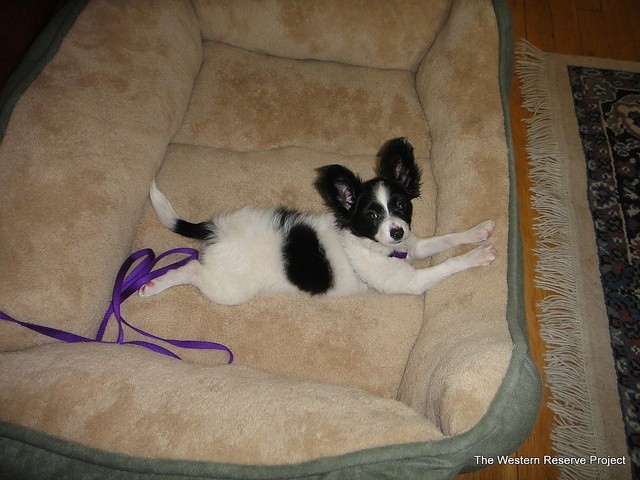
[136,135,498,307]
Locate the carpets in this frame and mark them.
[512,36,640,463]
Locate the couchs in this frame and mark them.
[1,0,545,480]
